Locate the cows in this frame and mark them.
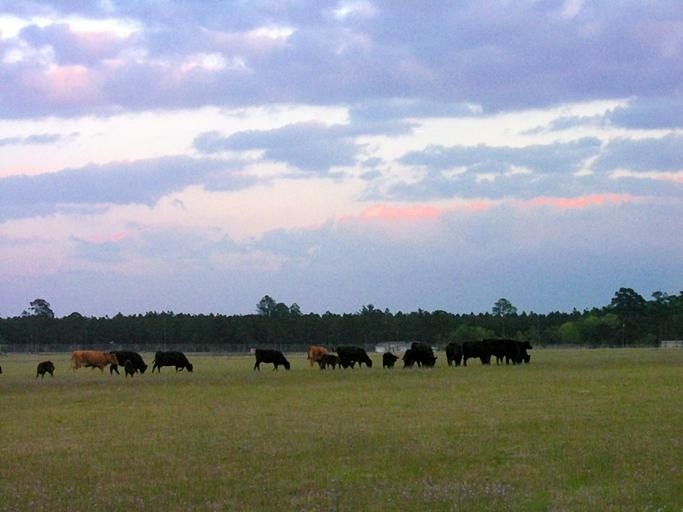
[401,342,437,369]
[306,344,372,372]
[36,360,55,379]
[445,342,462,368]
[462,337,533,368]
[253,348,290,372]
[70,350,148,378]
[382,352,399,368]
[152,350,193,373]
